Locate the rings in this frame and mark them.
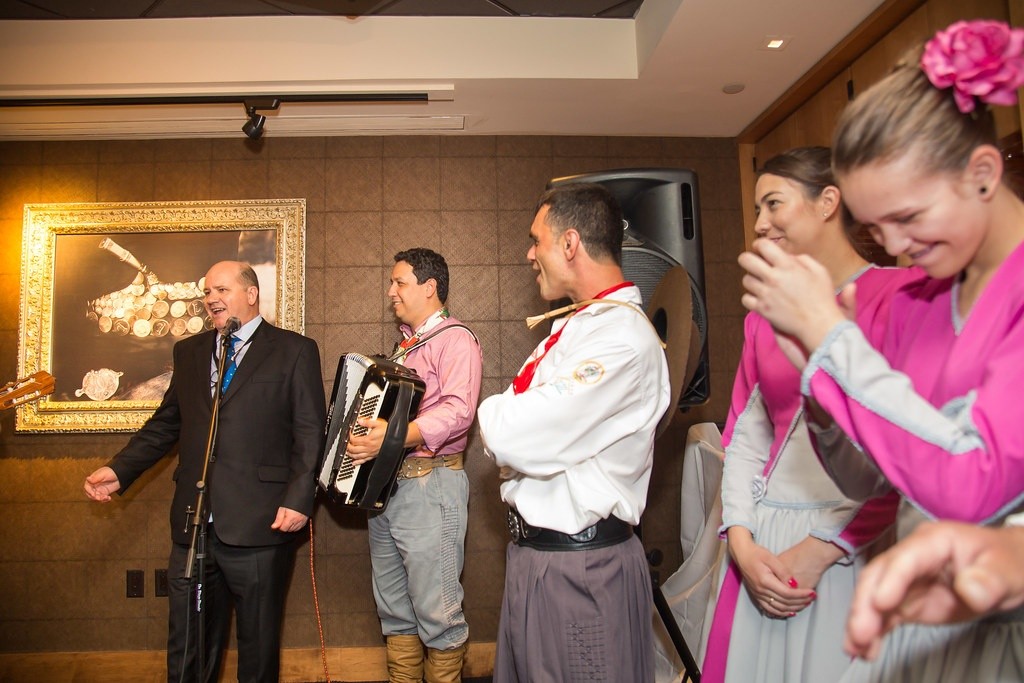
[768,598,775,604]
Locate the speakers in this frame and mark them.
[545,170,710,410]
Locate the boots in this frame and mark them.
[387,634,424,683]
[424,644,464,683]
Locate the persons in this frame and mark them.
[842,508,1024,664]
[736,22,1024,683]
[478,182,671,683]
[701,145,909,683]
[346,248,484,683]
[84,262,328,683]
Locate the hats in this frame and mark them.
[646,266,699,441]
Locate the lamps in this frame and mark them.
[242,98,280,139]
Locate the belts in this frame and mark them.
[507,509,635,552]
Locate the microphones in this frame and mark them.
[219,315,241,343]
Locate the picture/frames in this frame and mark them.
[15,198,305,433]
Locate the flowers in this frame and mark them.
[920,19,1024,114]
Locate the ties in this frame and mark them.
[220,338,242,393]
